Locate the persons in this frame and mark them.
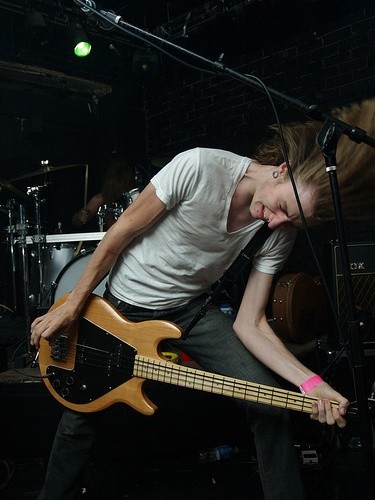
[72,160,137,233]
[28,100,375,500]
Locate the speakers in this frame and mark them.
[329,240,375,351]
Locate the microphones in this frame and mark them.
[211,222,272,304]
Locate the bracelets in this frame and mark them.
[299,374,325,395]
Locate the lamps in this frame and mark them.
[71,22,92,58]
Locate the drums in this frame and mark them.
[124,187,140,207]
[48,243,75,286]
[50,250,112,306]
[97,202,123,232]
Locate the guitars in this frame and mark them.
[38,292,341,421]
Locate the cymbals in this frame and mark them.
[26,215,52,231]
[0,205,17,217]
[7,161,81,184]
[0,175,32,202]
